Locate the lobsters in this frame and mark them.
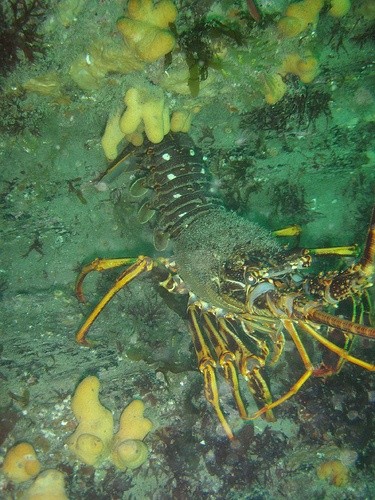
[73,129,374,438]
